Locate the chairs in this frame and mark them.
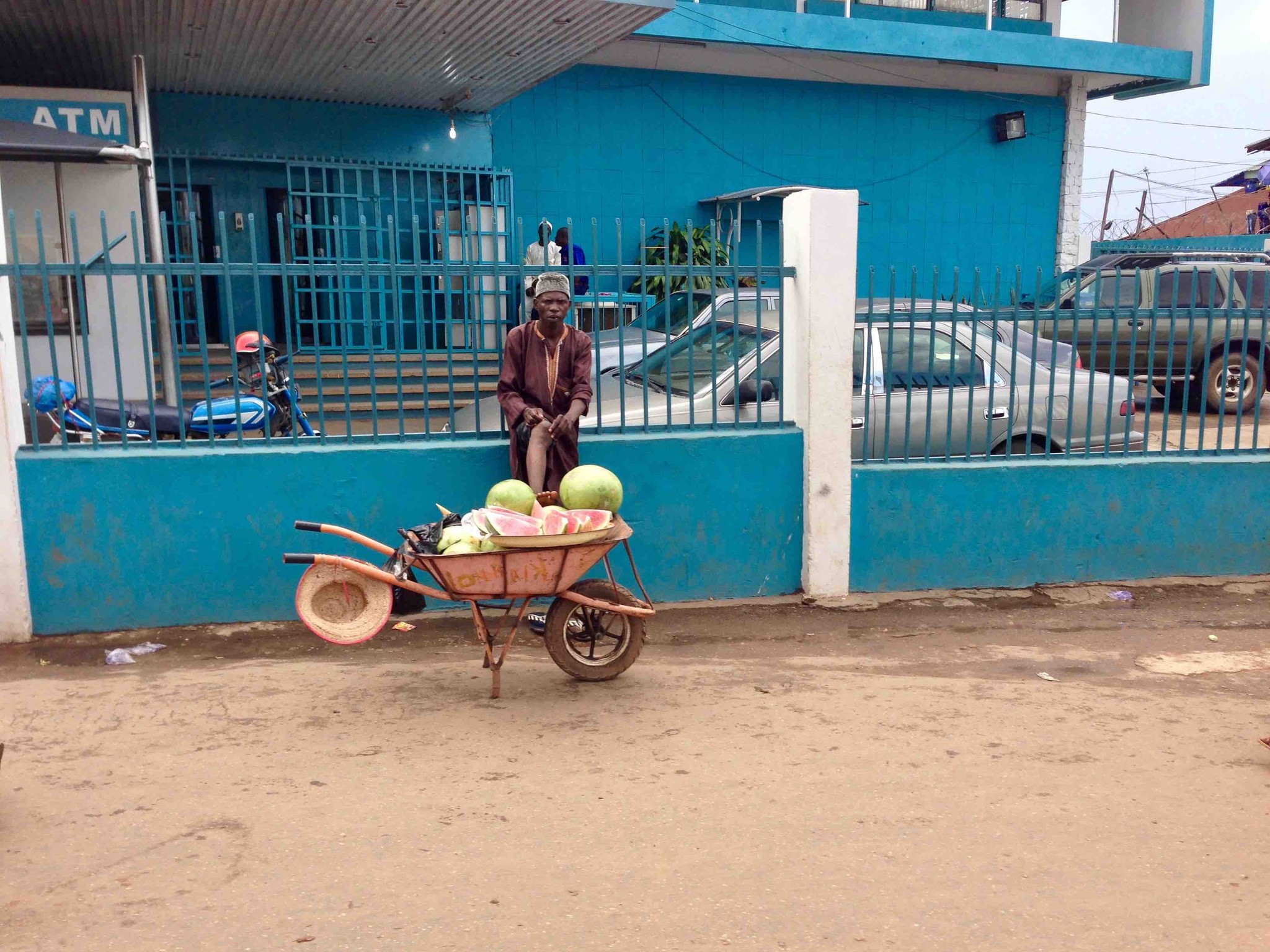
[902,338,935,359]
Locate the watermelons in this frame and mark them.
[437,464,624,555]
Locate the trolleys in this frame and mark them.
[281,511,658,700]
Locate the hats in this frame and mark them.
[294,557,393,645]
[539,221,552,231]
[535,272,570,299]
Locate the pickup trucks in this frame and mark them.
[989,251,1270,415]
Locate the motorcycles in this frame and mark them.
[43,348,327,445]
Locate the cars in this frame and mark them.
[443,307,1145,461]
[857,296,1083,372]
[586,286,779,380]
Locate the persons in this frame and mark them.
[518,220,561,325]
[554,227,589,296]
[1245,202,1270,234]
[497,272,593,641]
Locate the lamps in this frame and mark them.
[997,110,1025,143]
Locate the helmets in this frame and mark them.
[230,331,280,364]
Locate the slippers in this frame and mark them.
[567,619,591,641]
[527,612,547,634]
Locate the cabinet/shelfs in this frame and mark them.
[435,206,507,349]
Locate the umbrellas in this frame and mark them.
[1257,161,1270,185]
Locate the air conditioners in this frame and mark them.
[574,302,636,330]
